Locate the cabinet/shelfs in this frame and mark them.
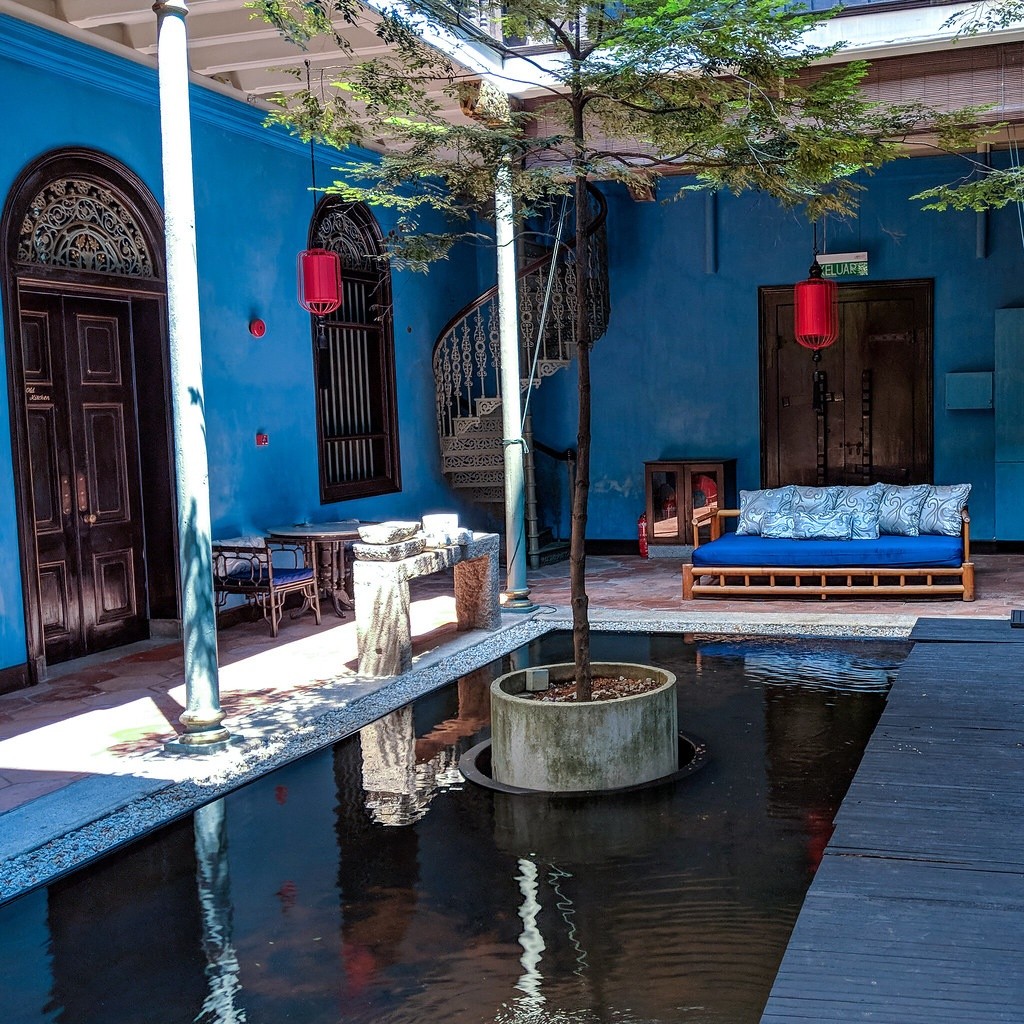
[644,461,737,545]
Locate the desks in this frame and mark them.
[269,522,371,620]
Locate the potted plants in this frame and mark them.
[247,0,1024,792]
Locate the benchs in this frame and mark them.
[681,482,976,603]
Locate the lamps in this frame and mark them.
[794,218,839,350]
[298,59,343,350]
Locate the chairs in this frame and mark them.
[212,536,322,638]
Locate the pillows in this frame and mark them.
[791,484,843,510]
[834,482,885,540]
[878,484,931,537]
[735,485,795,536]
[761,512,796,538]
[792,511,850,540]
[918,484,972,537]
[851,510,880,540]
